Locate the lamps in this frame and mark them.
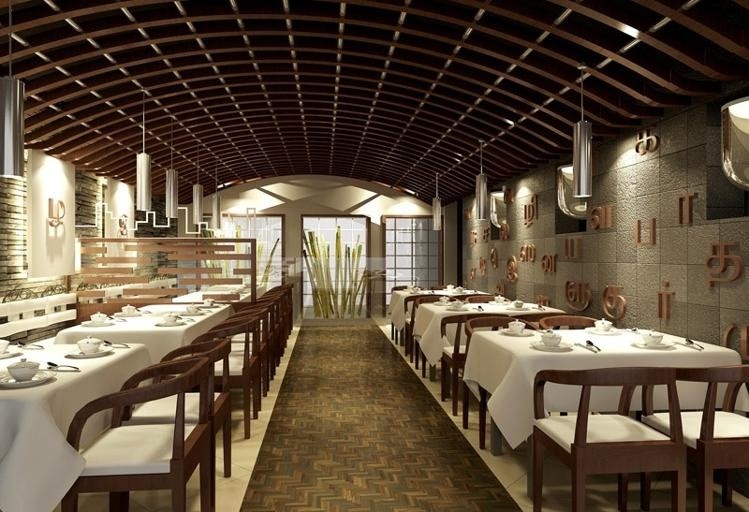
[490,192,506,229]
[193,142,204,224]
[721,96,749,192]
[213,168,220,229]
[557,164,586,220]
[48,199,66,226]
[120,215,128,235]
[476,139,488,220]
[433,173,442,230]
[572,64,592,196]
[0,0,25,178]
[166,118,178,218]
[136,91,151,211]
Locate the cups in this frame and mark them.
[594,317,613,331]
[406,284,524,309]
[644,329,664,344]
[541,331,561,345]
[509,319,525,332]
[0,339,9,354]
[8,358,40,380]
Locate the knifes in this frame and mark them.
[673,340,700,351]
[577,343,597,353]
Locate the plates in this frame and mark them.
[532,341,574,350]
[0,368,58,389]
[63,303,218,357]
[631,341,675,349]
[584,326,620,336]
[501,328,532,335]
[0,350,21,359]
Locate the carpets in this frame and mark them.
[372,314,749,512]
[55,327,300,512]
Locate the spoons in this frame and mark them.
[18,340,44,349]
[685,336,704,350]
[586,340,601,351]
[47,361,79,371]
